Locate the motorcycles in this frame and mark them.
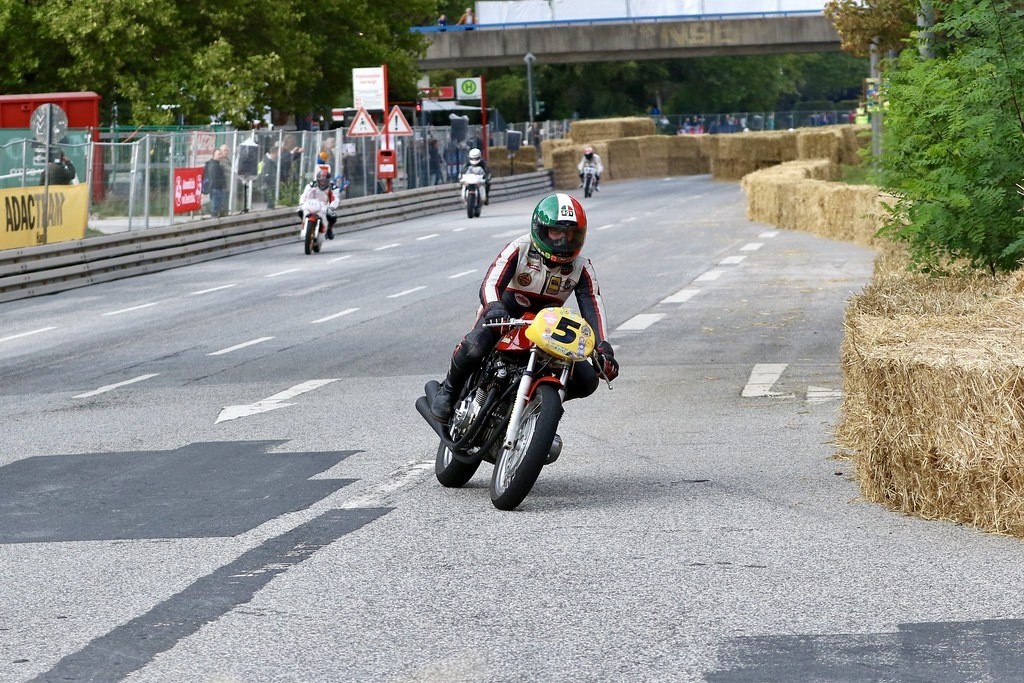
[578,162,599,198]
[412,305,613,514]
[457,165,492,219]
[296,186,330,255]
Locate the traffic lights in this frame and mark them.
[534,101,546,116]
[415,101,421,112]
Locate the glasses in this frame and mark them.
[549,227,575,236]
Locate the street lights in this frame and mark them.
[522,52,538,147]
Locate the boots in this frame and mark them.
[428,358,469,423]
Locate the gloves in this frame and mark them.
[485,301,508,324]
[594,341,618,381]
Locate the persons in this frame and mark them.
[429,194,618,423]
[39,149,76,186]
[201,109,870,239]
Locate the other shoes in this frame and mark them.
[327,228,334,239]
[579,183,583,188]
[484,197,489,205]
[595,187,600,192]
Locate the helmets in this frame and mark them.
[531,193,587,264]
[468,148,481,166]
[584,148,594,161]
[317,171,331,187]
